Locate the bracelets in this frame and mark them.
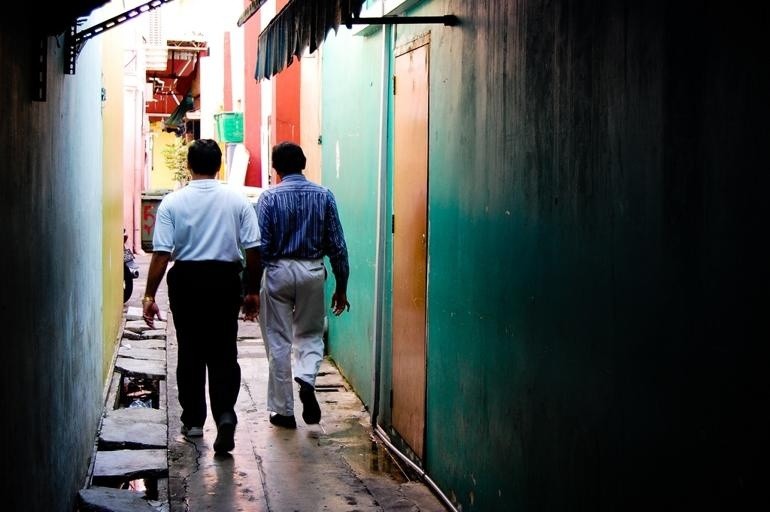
[141,296,156,304]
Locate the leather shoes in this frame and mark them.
[300,386,321,424]
[270,412,296,428]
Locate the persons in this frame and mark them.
[243,141,351,430]
[142,138,262,453]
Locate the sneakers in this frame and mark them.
[181,424,203,437]
[213,414,235,452]
